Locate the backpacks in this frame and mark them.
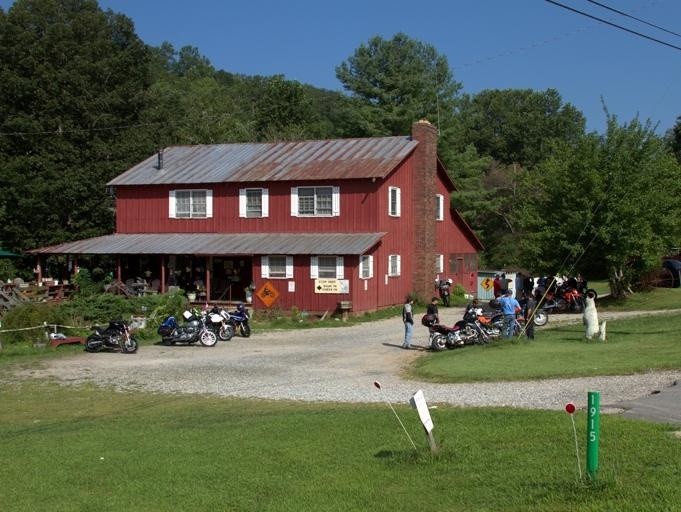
[158,316,178,336]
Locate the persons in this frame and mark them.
[400,272,583,349]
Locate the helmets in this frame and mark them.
[237,303,244,311]
[446,278,453,284]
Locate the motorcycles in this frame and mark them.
[261,291,275,298]
[84,317,139,354]
[221,303,253,338]
[159,304,219,350]
[421,269,603,352]
[183,305,235,343]
